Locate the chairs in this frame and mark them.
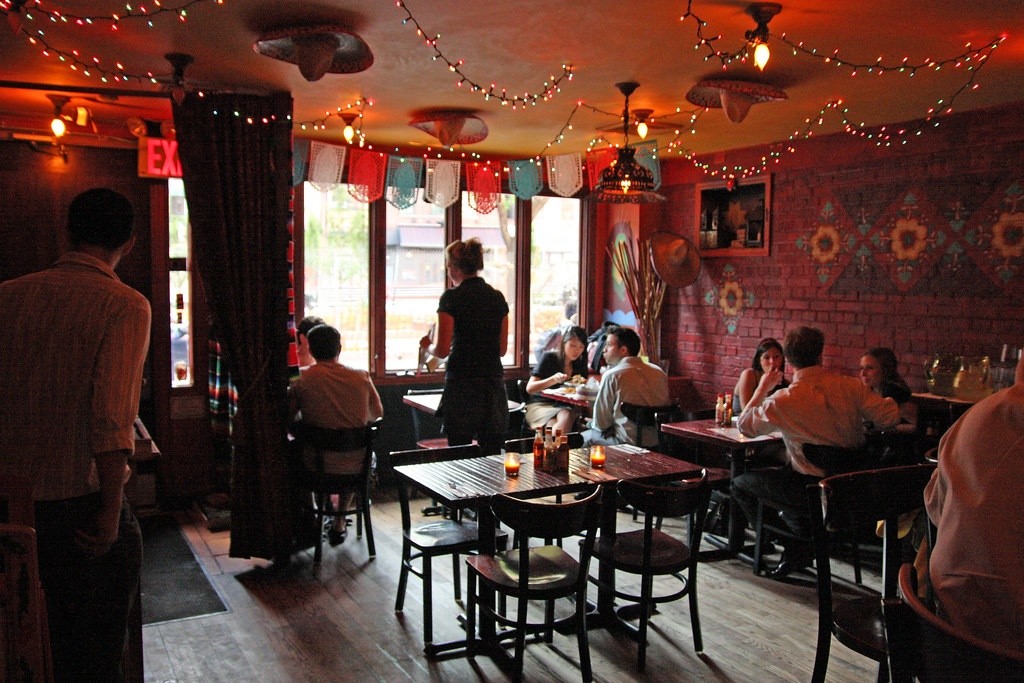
[293,379,1024,683]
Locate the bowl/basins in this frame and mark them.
[577,388,600,401]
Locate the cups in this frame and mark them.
[590,445,606,469]
[922,352,1018,402]
[504,452,520,476]
[175,362,187,380]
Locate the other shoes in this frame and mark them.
[330,522,348,543]
[766,551,812,579]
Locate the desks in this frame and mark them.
[392,352,971,671]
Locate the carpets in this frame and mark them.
[137,513,232,626]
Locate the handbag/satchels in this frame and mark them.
[700,492,748,536]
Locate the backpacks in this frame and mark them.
[586,319,620,371]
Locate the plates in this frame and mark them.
[563,382,586,387]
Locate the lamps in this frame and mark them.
[164,52,195,108]
[127,117,183,178]
[252,21,374,82]
[407,111,489,148]
[743,1,782,72]
[685,79,788,125]
[338,113,359,145]
[46,93,71,138]
[590,82,654,204]
[632,109,654,140]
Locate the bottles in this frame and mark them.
[715,394,724,425]
[533,426,570,473]
[723,394,732,426]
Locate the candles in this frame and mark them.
[505,452,519,472]
[590,445,605,465]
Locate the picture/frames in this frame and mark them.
[695,174,770,257]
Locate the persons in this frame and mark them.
[923,351,1024,653]
[731,326,918,580]
[287,315,383,545]
[0,186,152,683]
[525,297,670,510]
[419,237,509,529]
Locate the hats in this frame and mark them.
[647,231,700,288]
